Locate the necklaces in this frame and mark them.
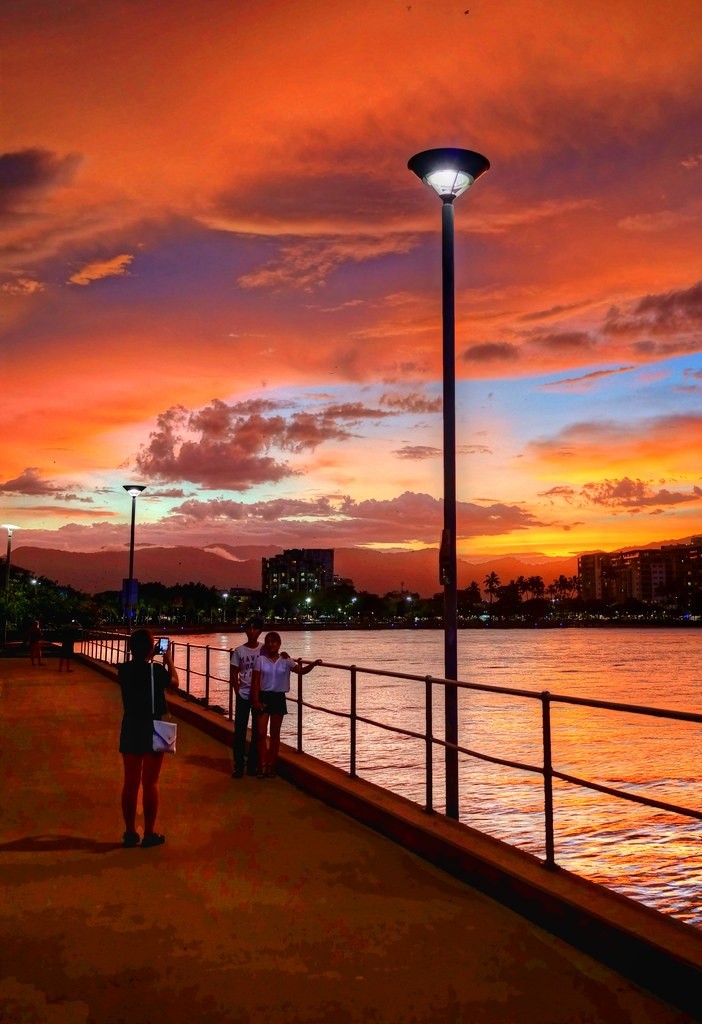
[266,654,274,663]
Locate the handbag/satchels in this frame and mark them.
[152,720,177,754]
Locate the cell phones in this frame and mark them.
[159,638,169,655]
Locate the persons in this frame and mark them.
[30,621,45,666]
[230,618,290,779]
[251,632,323,779]
[57,620,83,673]
[117,628,179,846]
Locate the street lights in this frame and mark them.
[1,523,18,643]
[408,150,493,821]
[123,485,147,664]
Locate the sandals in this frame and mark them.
[267,766,276,778]
[257,765,266,778]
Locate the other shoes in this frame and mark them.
[123,832,140,847]
[232,769,244,778]
[33,663,36,665]
[142,831,165,848]
[248,771,257,776]
[39,663,44,665]
[67,668,73,672]
[58,669,62,672]
